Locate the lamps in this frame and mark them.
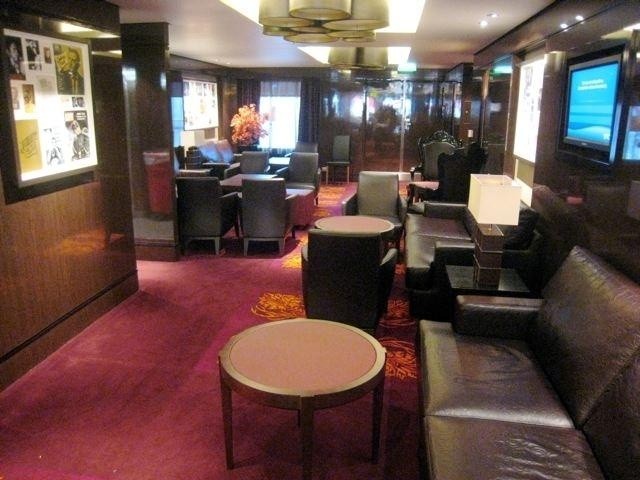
[323,44,392,73]
[462,172,523,287]
[468,128,475,140]
[247,0,395,51]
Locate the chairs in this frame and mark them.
[407,138,488,205]
[171,132,353,258]
[408,128,464,181]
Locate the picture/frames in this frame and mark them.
[0,24,101,190]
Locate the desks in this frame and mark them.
[219,312,392,480]
[312,214,395,258]
[441,260,535,324]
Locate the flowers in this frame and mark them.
[228,102,276,144]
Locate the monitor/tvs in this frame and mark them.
[555,43,629,167]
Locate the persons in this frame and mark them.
[66,49,86,94]
[68,119,90,156]
[12,88,18,108]
[46,51,50,61]
[28,40,37,66]
[4,39,22,76]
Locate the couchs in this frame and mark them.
[403,192,546,322]
[300,226,401,332]
[341,169,409,235]
[407,235,639,480]
